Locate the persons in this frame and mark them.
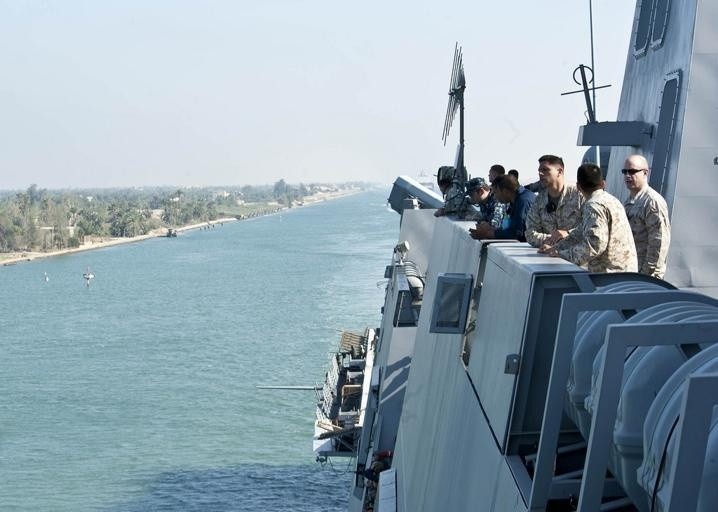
[508,170,525,193]
[537,161,637,273]
[461,177,495,223]
[432,166,482,221]
[488,164,504,187]
[620,154,670,279]
[468,174,534,243]
[522,154,583,247]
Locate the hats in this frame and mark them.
[462,177,485,196]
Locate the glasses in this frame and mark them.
[621,169,643,175]
